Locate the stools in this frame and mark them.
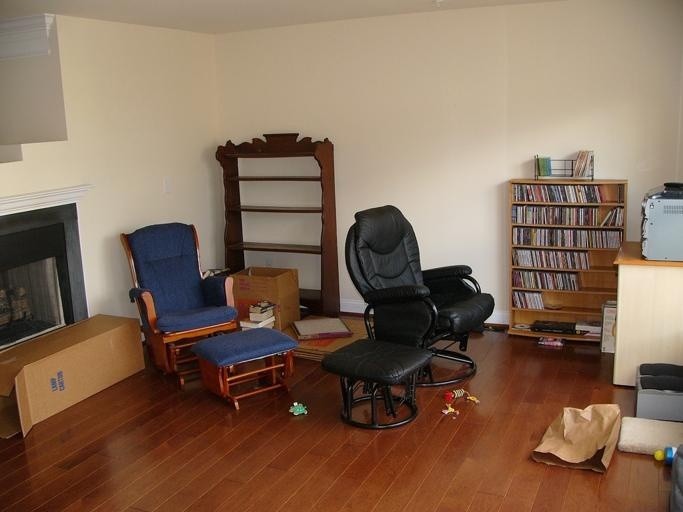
[190,325,295,410]
[321,338,435,431]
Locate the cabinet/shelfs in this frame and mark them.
[612,240,682,387]
[507,177,628,341]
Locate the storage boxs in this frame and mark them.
[0,311,143,443]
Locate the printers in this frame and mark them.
[640,180,683,262]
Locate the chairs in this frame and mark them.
[120,224,240,389]
[344,204,494,388]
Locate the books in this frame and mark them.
[239,303,276,331]
[511,150,624,346]
